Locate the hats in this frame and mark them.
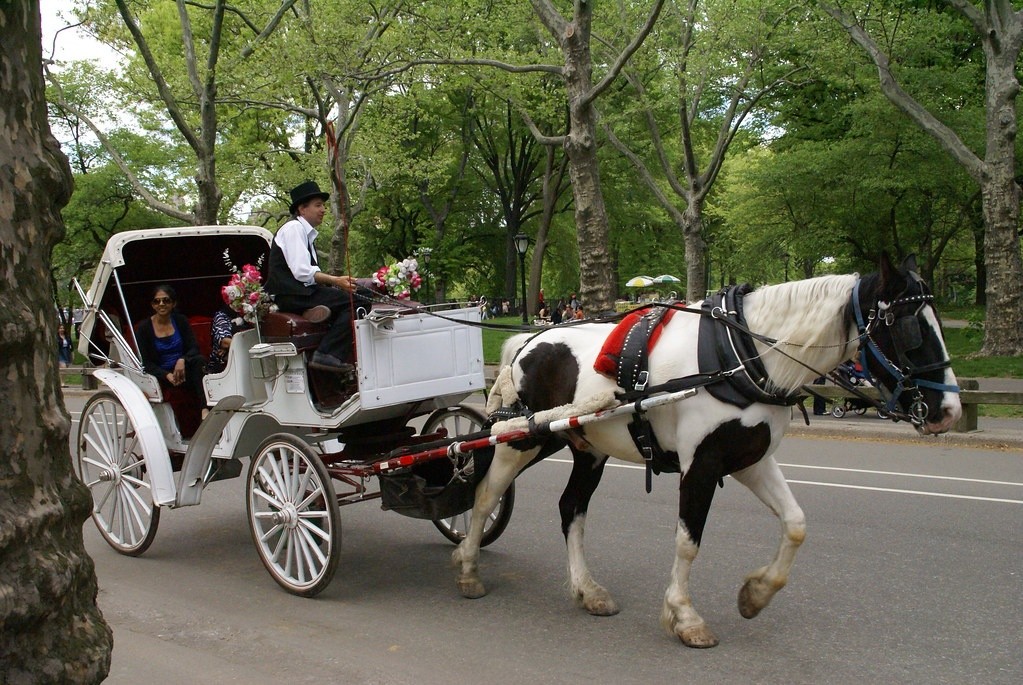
[289,182,330,215]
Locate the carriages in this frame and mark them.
[61,220,965,651]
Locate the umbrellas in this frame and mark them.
[625,274,681,287]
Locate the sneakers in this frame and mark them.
[308,351,354,372]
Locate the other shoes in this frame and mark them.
[61,385,69,388]
[202,412,209,420]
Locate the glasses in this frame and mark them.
[153,297,171,305]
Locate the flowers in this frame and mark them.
[372,246,435,301]
[221,247,278,330]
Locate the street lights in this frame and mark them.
[512,232,533,330]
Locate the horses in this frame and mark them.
[451,248,963,648]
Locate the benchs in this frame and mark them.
[125,315,215,428]
[257,276,427,341]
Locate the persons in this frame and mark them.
[269,181,372,407]
[536,288,584,325]
[58,324,74,388]
[623,289,660,302]
[813,374,831,416]
[449,294,509,320]
[136,286,255,420]
[854,351,880,387]
